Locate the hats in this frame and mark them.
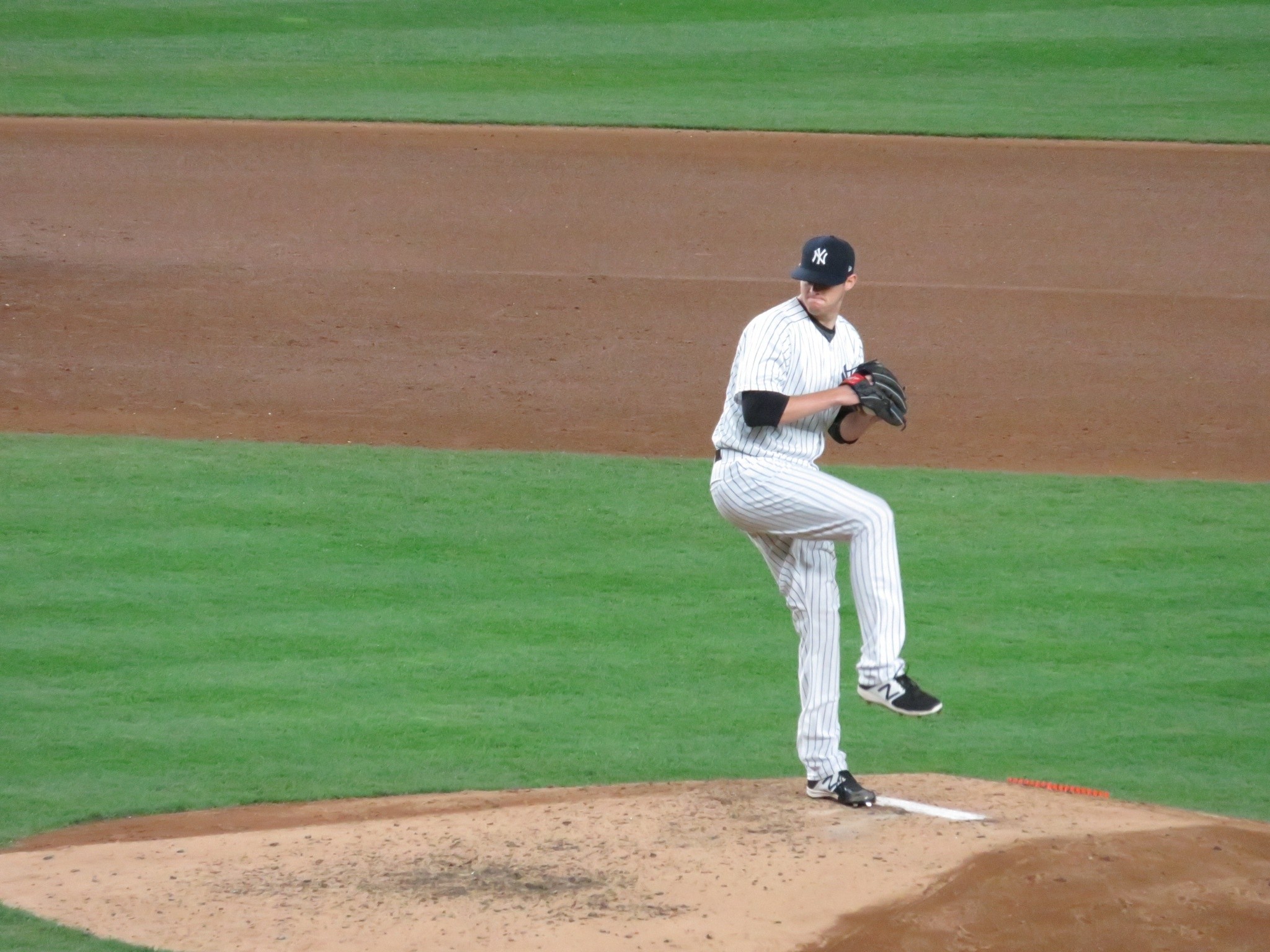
[791,235,855,285]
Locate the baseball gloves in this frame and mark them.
[839,361,907,426]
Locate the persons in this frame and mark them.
[709,236,944,807]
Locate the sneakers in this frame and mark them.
[806,769,874,807]
[856,673,942,718]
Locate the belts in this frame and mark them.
[715,449,721,462]
